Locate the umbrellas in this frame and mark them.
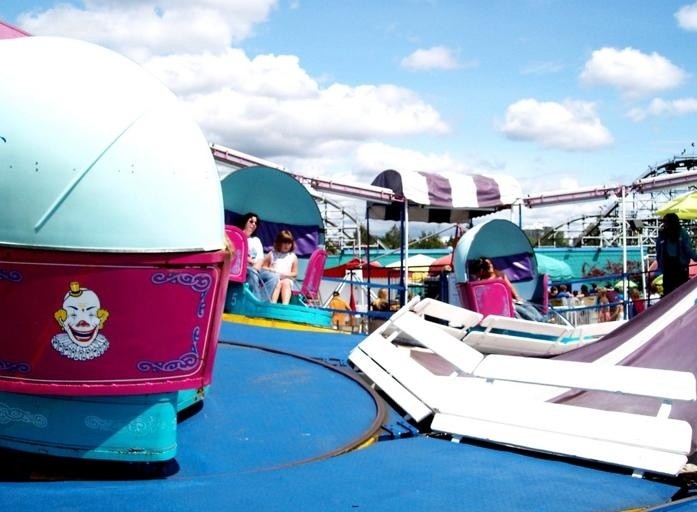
[656,189,697,220]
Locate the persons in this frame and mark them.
[261,230,298,305]
[477,258,556,324]
[242,211,264,300]
[327,291,354,331]
[548,281,663,323]
[654,213,697,296]
[371,288,424,333]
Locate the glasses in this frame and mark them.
[247,220,258,226]
